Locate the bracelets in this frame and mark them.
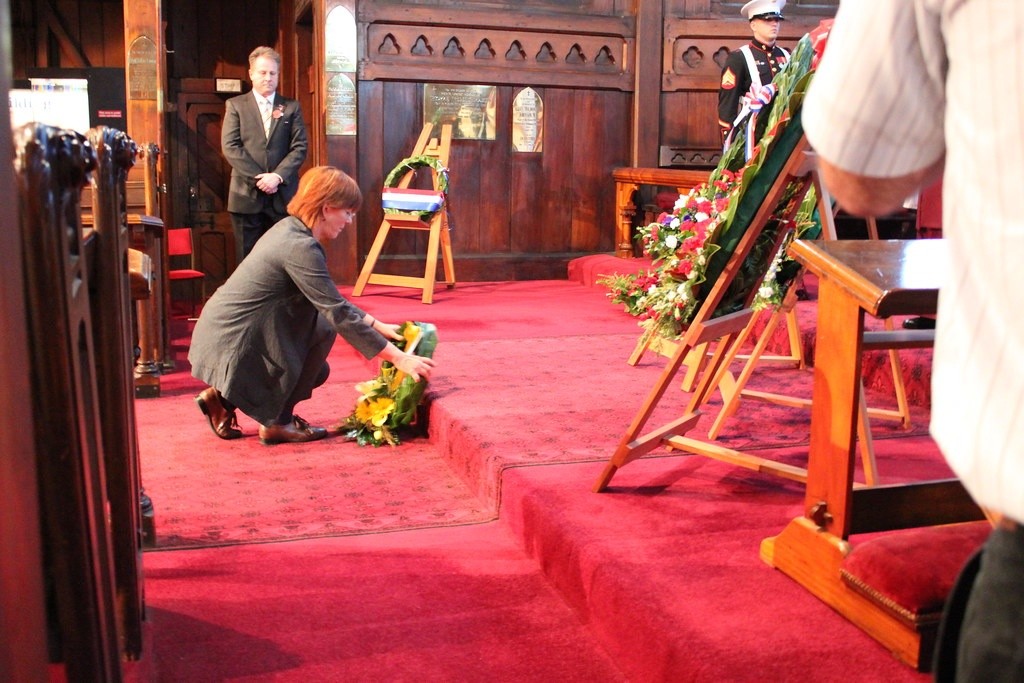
[371,319,376,327]
[279,178,281,183]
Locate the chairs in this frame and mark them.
[168,227,206,317]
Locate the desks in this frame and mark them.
[611,164,717,259]
[760,239,1016,669]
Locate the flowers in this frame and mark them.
[596,32,817,357]
[329,321,437,449]
[273,104,286,118]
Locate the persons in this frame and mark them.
[800,0,1023,683]
[188,165,438,445]
[717,0,794,173]
[222,47,308,265]
[902,181,942,330]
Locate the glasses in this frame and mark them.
[341,206,356,221]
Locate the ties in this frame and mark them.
[258,99,272,140]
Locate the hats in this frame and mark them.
[740,0,787,20]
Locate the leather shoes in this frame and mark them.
[260,415,329,444]
[195,386,242,440]
